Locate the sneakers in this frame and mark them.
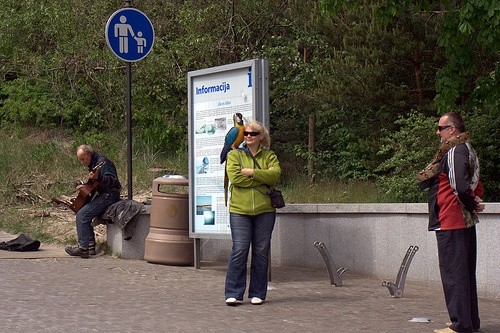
[88,245,96,256]
[64,244,90,259]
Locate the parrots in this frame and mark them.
[220,113,245,207]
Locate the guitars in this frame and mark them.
[70,160,105,214]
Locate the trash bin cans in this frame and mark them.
[143,175,201,266]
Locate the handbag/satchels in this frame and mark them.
[269,189,286,209]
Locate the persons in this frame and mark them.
[426,111,486,333]
[64,144,123,259]
[223,120,282,305]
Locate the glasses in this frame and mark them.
[435,125,455,132]
[243,130,260,136]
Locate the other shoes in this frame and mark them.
[250,296,264,305]
[226,297,237,305]
[433,321,481,333]
